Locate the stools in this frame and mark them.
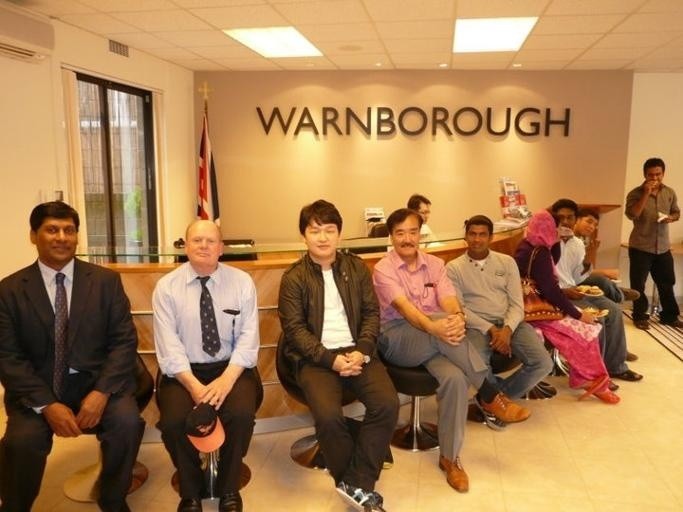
[469,352,521,423]
[62,353,154,501]
[156,364,263,500]
[276,332,358,468]
[520,337,557,399]
[378,350,440,452]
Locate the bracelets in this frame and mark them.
[456,312,466,319]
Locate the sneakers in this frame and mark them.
[336,478,386,512]
[472,394,506,431]
[369,493,382,509]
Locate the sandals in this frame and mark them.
[616,368,642,381]
[598,387,619,404]
[609,380,619,391]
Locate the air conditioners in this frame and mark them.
[0,0,55,64]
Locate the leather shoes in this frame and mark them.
[218,489,243,512]
[625,352,637,361]
[618,287,641,301]
[439,455,469,493]
[479,392,531,423]
[177,495,203,512]
[634,318,649,330]
[96,494,131,511]
[660,315,682,327]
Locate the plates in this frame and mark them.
[581,290,604,297]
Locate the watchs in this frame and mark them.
[362,353,371,368]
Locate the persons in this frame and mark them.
[1,201,145,512]
[523,210,620,403]
[387,195,441,251]
[574,209,639,361]
[373,208,532,493]
[446,215,554,431]
[624,158,682,330]
[278,199,400,511]
[149,219,260,512]
[549,198,643,380]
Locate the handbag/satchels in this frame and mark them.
[521,245,564,322]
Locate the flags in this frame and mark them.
[197,113,220,228]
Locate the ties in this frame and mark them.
[196,275,221,357]
[52,272,69,403]
